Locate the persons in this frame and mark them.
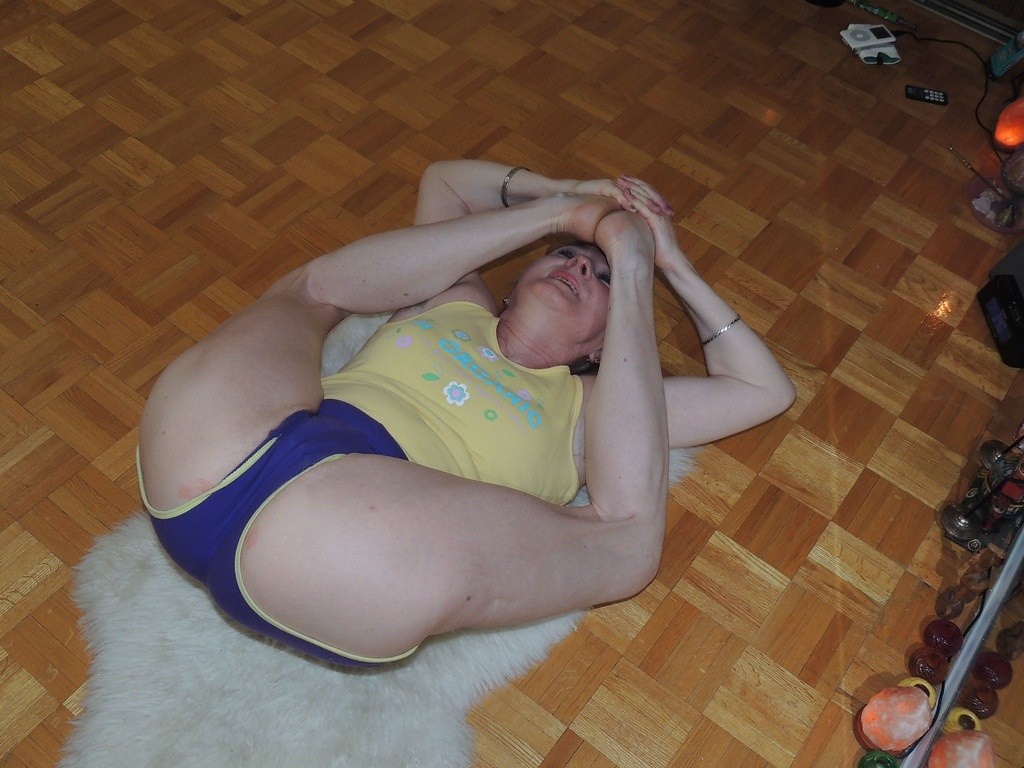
[137,160,797,666]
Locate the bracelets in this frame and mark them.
[502,165,530,208]
[703,314,740,346]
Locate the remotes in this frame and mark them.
[905,85,949,106]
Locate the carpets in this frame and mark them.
[62,315,702,768]
